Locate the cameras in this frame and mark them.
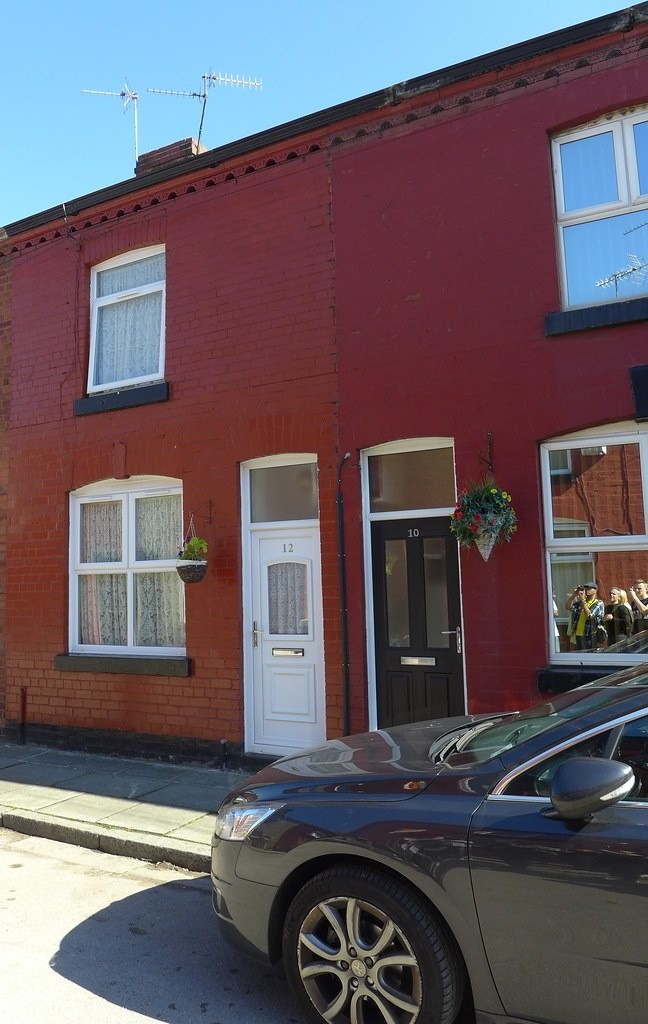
[577,586,584,592]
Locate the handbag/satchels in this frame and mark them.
[615,634,627,643]
[593,600,608,643]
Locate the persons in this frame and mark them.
[552,587,560,653]
[565,579,648,651]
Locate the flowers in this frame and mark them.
[176,535,208,561]
[449,469,518,549]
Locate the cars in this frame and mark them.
[209,657,648,1024]
[559,626,648,656]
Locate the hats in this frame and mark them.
[584,582,598,590]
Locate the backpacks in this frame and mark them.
[610,602,633,624]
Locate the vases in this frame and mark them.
[176,560,208,584]
[475,514,505,561]
